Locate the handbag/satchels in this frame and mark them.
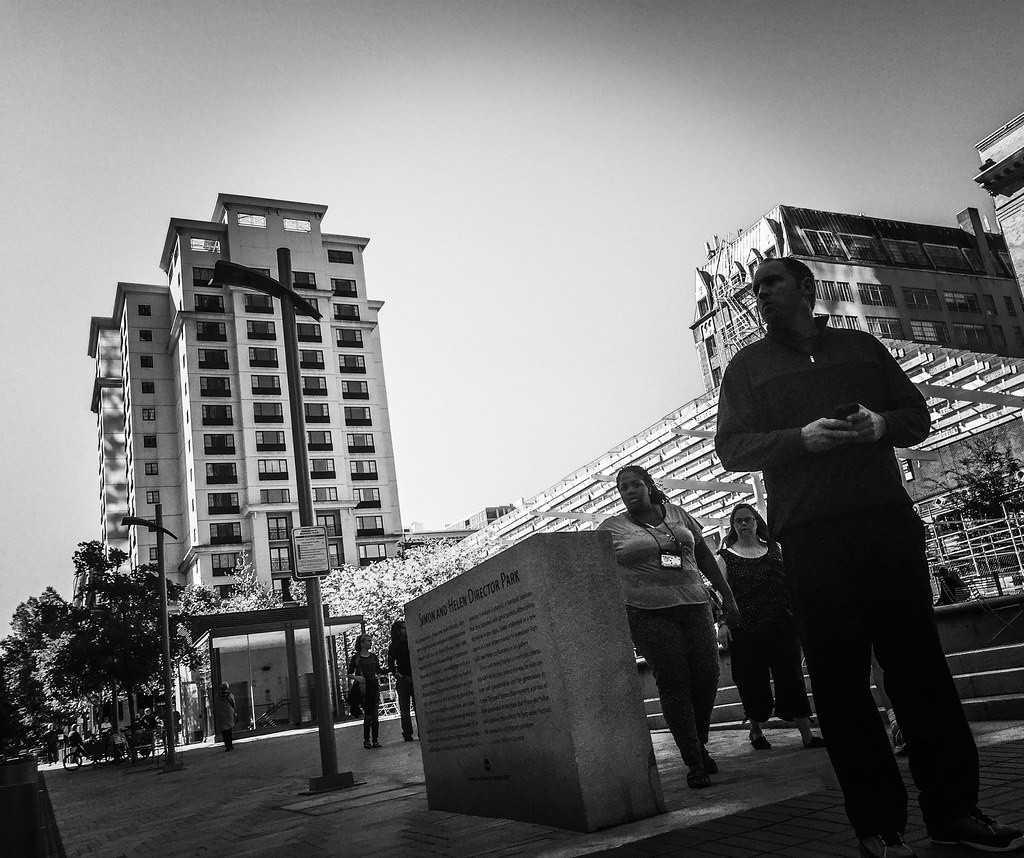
[232,710,239,724]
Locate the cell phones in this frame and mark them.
[834,403,859,424]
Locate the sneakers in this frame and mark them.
[923,803,1023,852]
[860,834,915,858]
[891,725,910,756]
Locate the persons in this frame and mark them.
[172,706,181,744]
[711,504,825,751]
[41,722,59,766]
[347,634,398,749]
[715,255,1024,858]
[214,681,237,752]
[597,466,741,790]
[139,707,158,744]
[388,621,420,741]
[155,716,164,733]
[100,717,112,739]
[68,724,84,746]
[933,567,964,608]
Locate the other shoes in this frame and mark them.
[403,733,414,742]
[746,732,771,750]
[225,746,235,752]
[362,740,382,748]
[800,737,825,749]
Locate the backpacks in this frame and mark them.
[347,678,370,706]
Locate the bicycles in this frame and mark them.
[62,729,115,772]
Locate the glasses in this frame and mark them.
[731,516,757,525]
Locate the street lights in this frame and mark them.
[213,247,368,795]
[120,505,188,774]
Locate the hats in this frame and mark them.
[930,565,949,575]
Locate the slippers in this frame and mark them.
[684,768,710,788]
[703,756,719,775]
